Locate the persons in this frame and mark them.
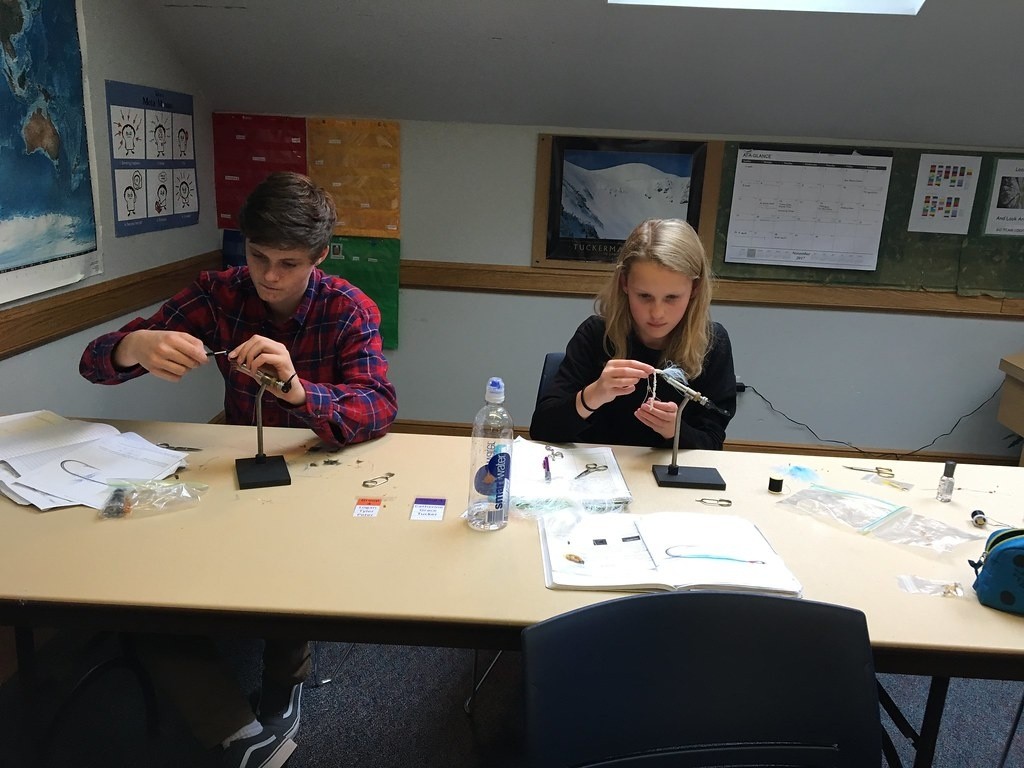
[80,173,399,768]
[529,218,736,449]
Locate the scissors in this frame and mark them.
[844,465,895,478]
[574,462,608,479]
[157,441,204,453]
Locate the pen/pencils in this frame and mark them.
[542,457,551,482]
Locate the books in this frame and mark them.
[536,507,803,599]
[509,435,633,508]
[0,408,189,512]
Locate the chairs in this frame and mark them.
[525,591,880,768]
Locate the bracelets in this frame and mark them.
[581,386,597,412]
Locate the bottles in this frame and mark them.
[936,461,957,502]
[468,377,514,532]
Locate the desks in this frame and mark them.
[0,413,1024,768]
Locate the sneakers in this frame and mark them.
[214,680,301,768]
